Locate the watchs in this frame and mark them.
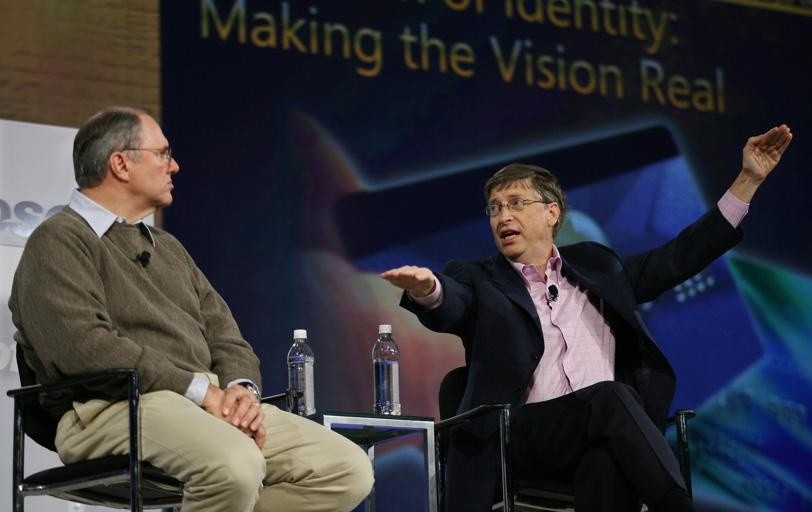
[238,383,260,401]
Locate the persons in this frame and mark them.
[8,106,375,512]
[380,124,794,512]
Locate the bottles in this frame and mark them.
[286,327,318,416]
[371,323,403,419]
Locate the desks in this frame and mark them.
[305,409,438,511]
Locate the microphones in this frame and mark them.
[549,284,558,297]
[141,250,150,267]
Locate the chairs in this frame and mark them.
[435,363,698,512]
[7,330,306,511]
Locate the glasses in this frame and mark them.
[123,143,174,160]
[485,198,551,216]
